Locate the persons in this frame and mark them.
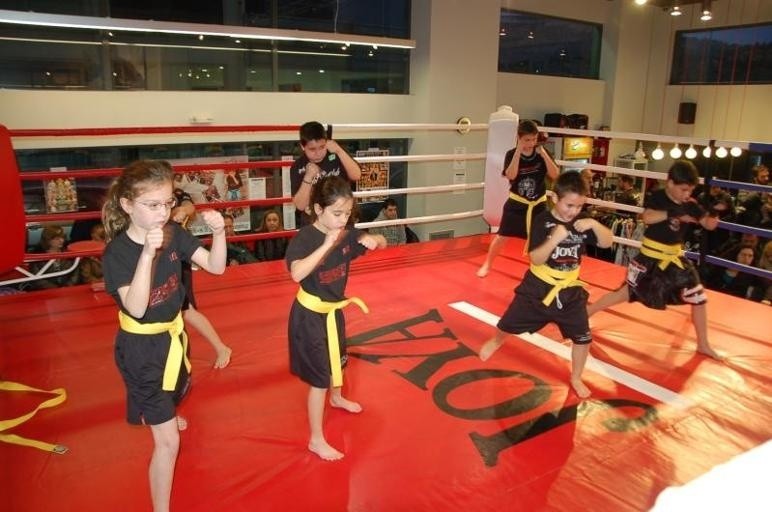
[349,199,407,246]
[283,173,388,464]
[584,158,730,365]
[474,117,562,280]
[577,155,772,306]
[478,169,616,399]
[27,191,130,289]
[172,187,234,371]
[170,190,290,270]
[292,119,366,230]
[98,157,229,512]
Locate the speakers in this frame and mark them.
[678,103,696,124]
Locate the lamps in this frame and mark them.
[664,0,718,23]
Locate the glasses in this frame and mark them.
[134,198,176,211]
